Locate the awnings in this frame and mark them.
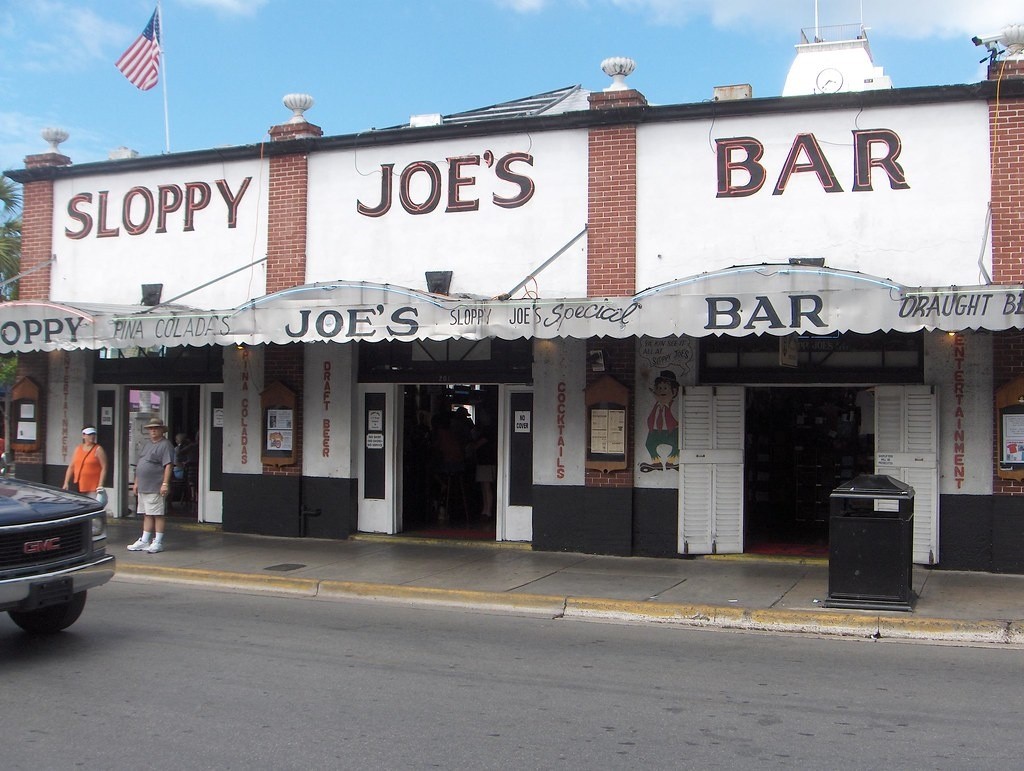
[0,206,1024,357]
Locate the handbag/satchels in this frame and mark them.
[69,480,79,492]
[95,487,108,507]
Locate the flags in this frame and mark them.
[115,7,161,91]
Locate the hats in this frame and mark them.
[80,427,98,435]
[143,417,169,434]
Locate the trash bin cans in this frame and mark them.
[822,473,922,613]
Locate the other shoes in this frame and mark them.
[92,530,107,542]
[438,511,449,520]
[475,512,492,522]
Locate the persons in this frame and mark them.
[0,434,4,468]
[126,419,174,552]
[409,405,498,522]
[174,426,199,503]
[63,424,108,500]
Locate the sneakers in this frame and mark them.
[127,537,151,551]
[147,538,164,553]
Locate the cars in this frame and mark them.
[0,452,116,634]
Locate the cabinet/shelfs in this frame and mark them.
[787,423,838,536]
